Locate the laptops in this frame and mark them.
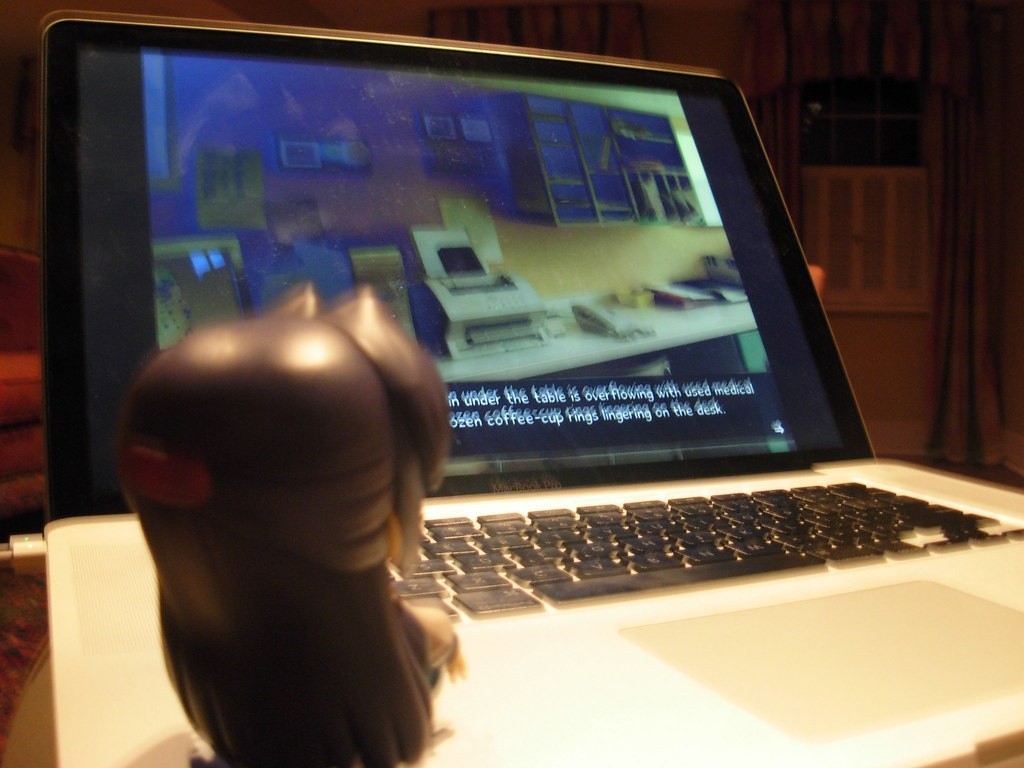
[35,18,1024,768]
